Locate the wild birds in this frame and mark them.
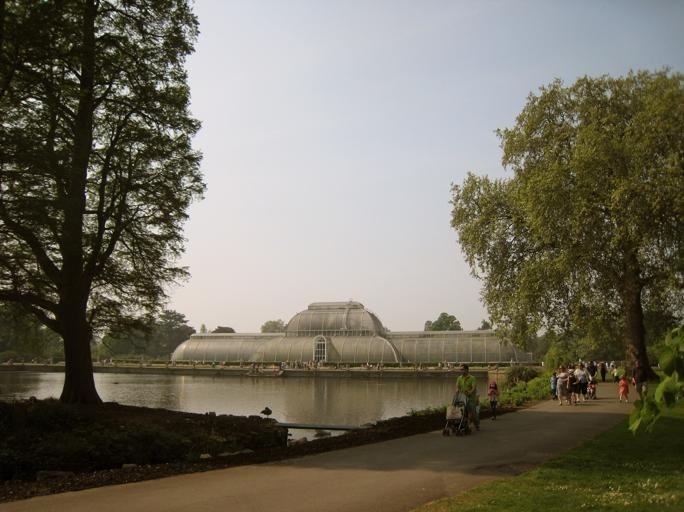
[259,406,272,418]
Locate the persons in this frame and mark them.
[487,380,501,420]
[550,360,635,407]
[467,385,480,432]
[454,364,481,432]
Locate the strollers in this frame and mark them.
[442,390,472,438]
[587,381,597,400]
